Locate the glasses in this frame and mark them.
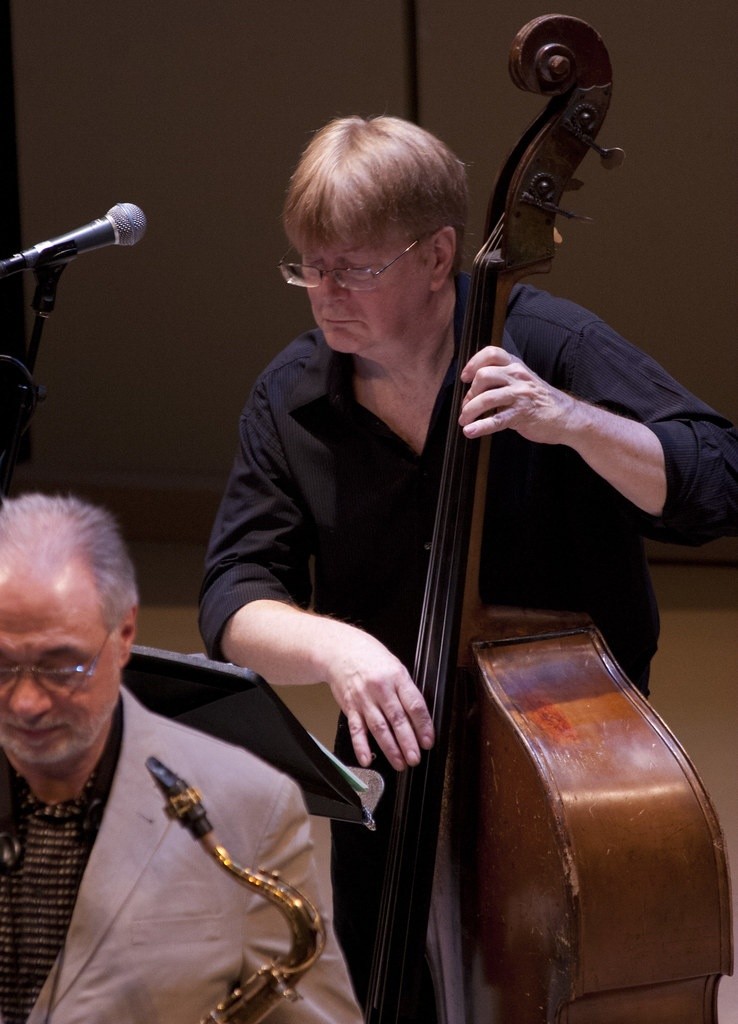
[275,233,432,292]
[0,630,118,695]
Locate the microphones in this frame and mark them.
[0,202,147,280]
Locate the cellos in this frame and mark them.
[360,15,731,1024]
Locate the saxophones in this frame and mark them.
[138,751,328,1023]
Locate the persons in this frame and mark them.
[198,118,738,1023]
[0,494,365,1024]
